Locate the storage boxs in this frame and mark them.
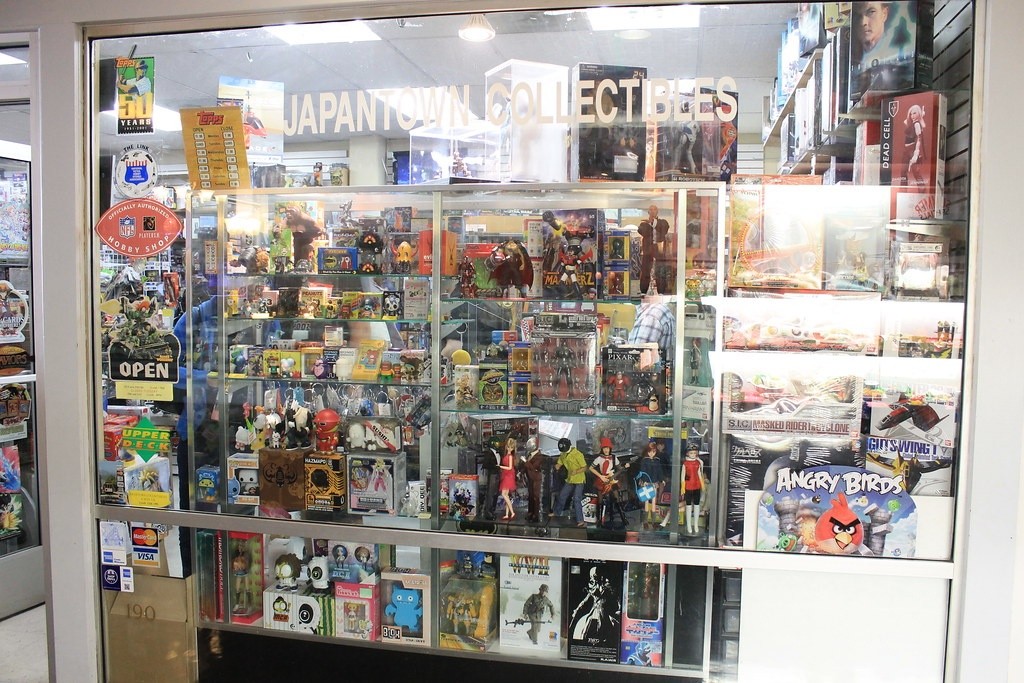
[762,1,947,221]
[334,582,380,643]
[380,567,431,647]
[440,549,707,672]
[656,87,739,180]
[197,464,220,504]
[262,579,309,633]
[105,405,183,511]
[326,539,391,585]
[569,63,648,181]
[295,579,336,637]
[100,567,207,683]
[227,207,706,549]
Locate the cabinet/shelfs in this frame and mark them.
[182,182,729,679]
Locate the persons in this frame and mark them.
[629,303,675,361]
[637,205,669,295]
[688,338,703,385]
[851,1,890,67]
[482,437,671,530]
[680,442,705,534]
[550,341,578,401]
[543,209,595,301]
[522,583,555,645]
[900,105,926,192]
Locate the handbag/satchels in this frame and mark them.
[154,387,184,415]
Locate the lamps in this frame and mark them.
[456,14,495,43]
